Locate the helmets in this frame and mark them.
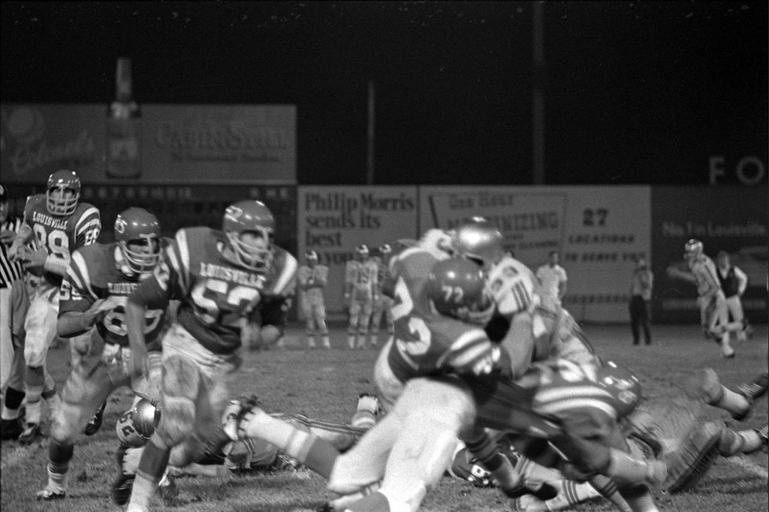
[356,244,370,259]
[113,205,165,275]
[450,216,510,264]
[594,359,645,420]
[378,242,393,256]
[306,249,319,265]
[131,398,161,438]
[46,167,83,218]
[425,257,497,327]
[219,198,278,275]
[682,238,703,260]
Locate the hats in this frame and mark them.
[0,182,11,202]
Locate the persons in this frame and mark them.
[0,170,768,511]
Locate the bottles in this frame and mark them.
[105,58,141,179]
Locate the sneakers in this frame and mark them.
[109,441,145,506]
[190,393,261,462]
[744,423,769,456]
[501,476,560,503]
[358,390,389,422]
[17,424,40,448]
[84,398,107,436]
[742,318,756,343]
[35,484,66,503]
[729,373,769,422]
